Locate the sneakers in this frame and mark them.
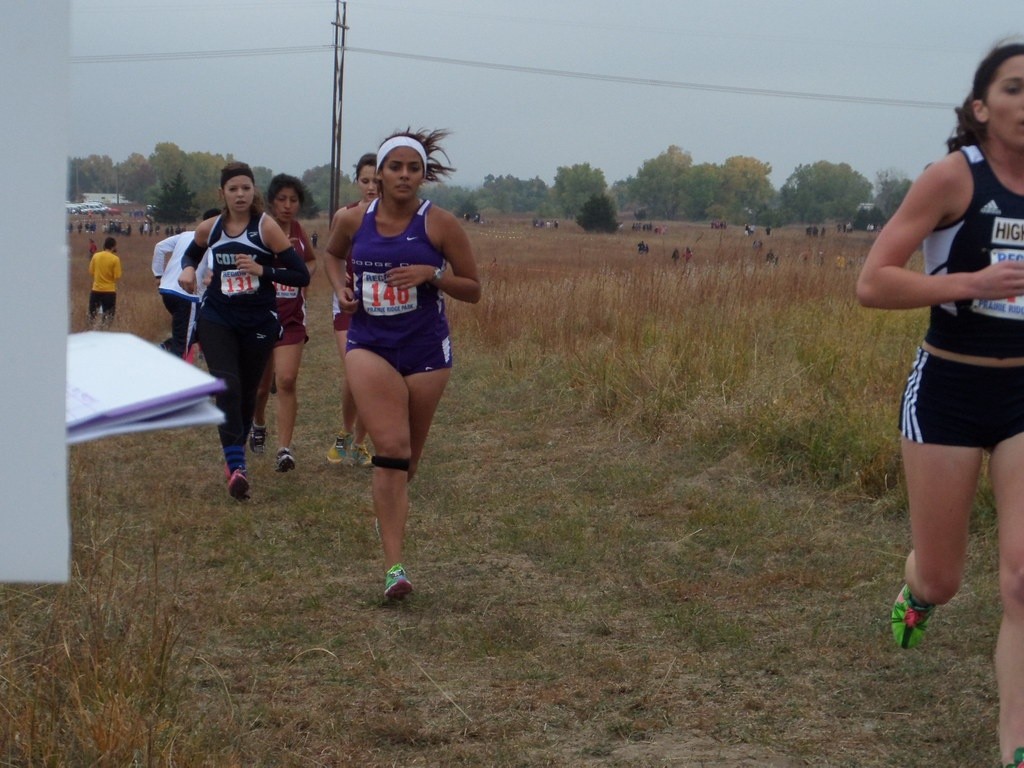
[248,417,267,455]
[891,584,939,649]
[384,563,413,600]
[182,342,202,364]
[326,432,355,464]
[351,444,373,467]
[225,462,232,480]
[228,468,251,502]
[271,372,277,394]
[274,446,296,473]
[374,516,380,540]
[1006,747,1024,768]
[158,343,171,351]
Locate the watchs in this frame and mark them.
[431,266,442,280]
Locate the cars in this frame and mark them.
[66,203,110,215]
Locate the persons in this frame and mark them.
[633,222,666,234]
[68,222,96,234]
[710,219,727,229]
[88,236,122,322]
[102,219,131,235]
[638,241,649,253]
[532,218,558,228]
[150,161,317,503]
[139,220,186,237]
[464,210,480,223]
[323,132,482,600]
[311,230,319,248]
[858,44,1024,768]
[866,223,882,232]
[672,247,692,263]
[805,221,856,239]
[744,223,772,237]
[328,153,383,466]
